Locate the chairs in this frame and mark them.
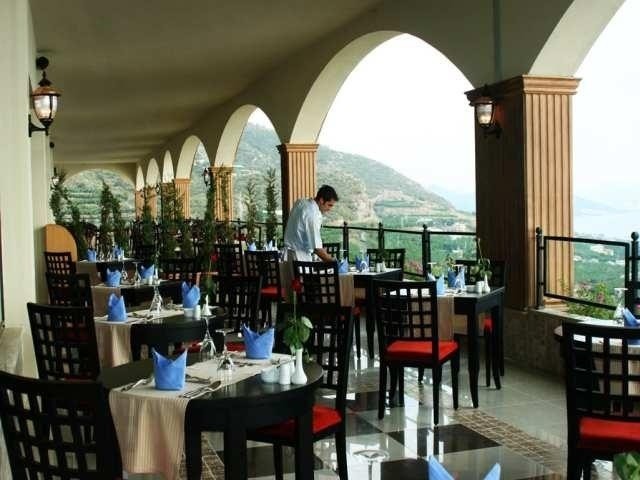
[211,275,258,352]
[0,370,108,480]
[214,243,244,329]
[366,249,404,278]
[244,249,285,330]
[45,272,94,343]
[375,279,460,425]
[27,302,100,442]
[455,258,506,387]
[293,259,361,359]
[43,251,73,274]
[560,319,640,480]
[246,303,354,480]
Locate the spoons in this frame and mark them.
[187,380,221,398]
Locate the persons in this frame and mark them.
[283,184,339,283]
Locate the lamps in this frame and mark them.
[50,173,60,190]
[469,83,502,139]
[155,184,160,195]
[28,70,62,138]
[201,168,211,187]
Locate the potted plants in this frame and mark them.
[377,248,390,272]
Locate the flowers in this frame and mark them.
[283,280,313,364]
[472,237,492,280]
[200,252,220,309]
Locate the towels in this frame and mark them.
[264,240,272,251]
[108,294,126,321]
[152,347,188,388]
[427,272,445,295]
[182,282,200,308]
[88,249,96,261]
[447,267,465,289]
[624,307,640,345]
[333,256,348,272]
[429,455,500,480]
[247,241,256,250]
[106,268,122,286]
[140,264,154,284]
[356,255,369,270]
[243,324,274,358]
[113,247,120,258]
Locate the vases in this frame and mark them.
[202,294,212,316]
[482,274,490,292]
[291,348,307,385]
[382,260,386,272]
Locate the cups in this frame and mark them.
[262,366,280,383]
[150,281,164,311]
[215,328,236,382]
[184,308,194,318]
[120,259,128,279]
[613,286,627,326]
[339,249,347,262]
[359,249,369,272]
[427,261,437,282]
[454,264,465,296]
[200,315,216,361]
[133,261,141,283]
[370,267,375,272]
[467,285,476,292]
